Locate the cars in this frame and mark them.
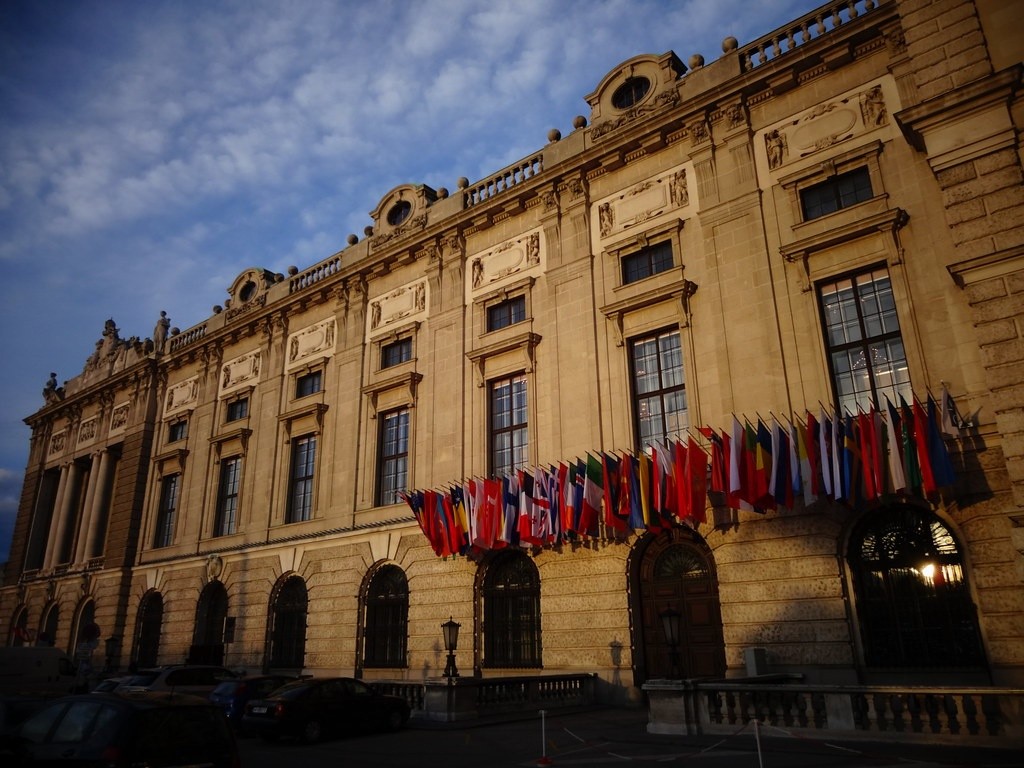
[0,689,245,766]
[211,675,304,737]
[249,677,414,747]
[0,647,91,736]
[96,671,172,712]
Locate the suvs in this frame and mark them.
[115,664,242,722]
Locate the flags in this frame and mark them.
[400,379,979,559]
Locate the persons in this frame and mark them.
[30,651,142,695]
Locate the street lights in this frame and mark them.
[440,615,460,721]
